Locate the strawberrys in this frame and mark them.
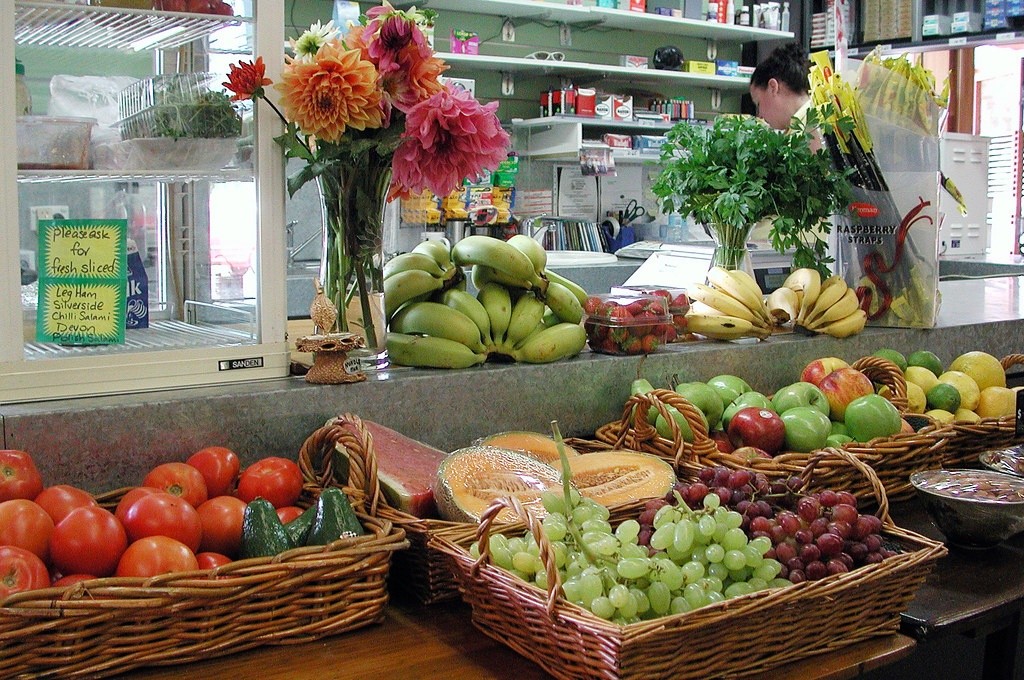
[584,290,692,356]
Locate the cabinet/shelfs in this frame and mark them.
[362,0,796,163]
[0,0,291,402]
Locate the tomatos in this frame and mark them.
[0,446,305,605]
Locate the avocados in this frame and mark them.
[232,484,365,560]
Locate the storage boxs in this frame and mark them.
[108,65,251,137]
[619,55,649,70]
[716,60,738,76]
[584,281,694,357]
[617,0,646,13]
[680,59,715,75]
[540,88,634,147]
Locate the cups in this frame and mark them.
[471,227,493,237]
[420,232,450,252]
[445,218,472,246]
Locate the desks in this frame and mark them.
[114,579,916,680]
[862,501,1024,680]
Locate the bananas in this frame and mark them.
[383,233,588,369]
[686,267,775,342]
[764,267,868,338]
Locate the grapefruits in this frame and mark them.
[946,350,1017,419]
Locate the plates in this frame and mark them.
[90,139,240,170]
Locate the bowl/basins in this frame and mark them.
[978,447,1024,477]
[17,115,98,170]
[611,285,693,343]
[910,469,1024,549]
[583,294,674,356]
[109,74,242,141]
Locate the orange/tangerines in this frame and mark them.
[864,350,981,426]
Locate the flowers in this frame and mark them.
[221,0,514,202]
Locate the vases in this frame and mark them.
[703,220,757,342]
[307,165,397,380]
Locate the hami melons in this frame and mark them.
[431,430,676,525]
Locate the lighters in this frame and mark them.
[648,98,695,119]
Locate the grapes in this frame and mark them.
[472,466,900,625]
[922,450,1024,503]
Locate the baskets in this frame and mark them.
[0,427,410,679]
[326,358,1024,679]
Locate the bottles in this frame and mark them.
[15,56,32,116]
[734,6,750,26]
[649,99,694,118]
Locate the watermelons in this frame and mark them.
[328,420,454,517]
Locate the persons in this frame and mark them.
[750,42,822,155]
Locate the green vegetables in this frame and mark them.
[121,74,242,139]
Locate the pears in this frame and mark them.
[628,369,724,444]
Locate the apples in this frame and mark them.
[705,355,917,459]
